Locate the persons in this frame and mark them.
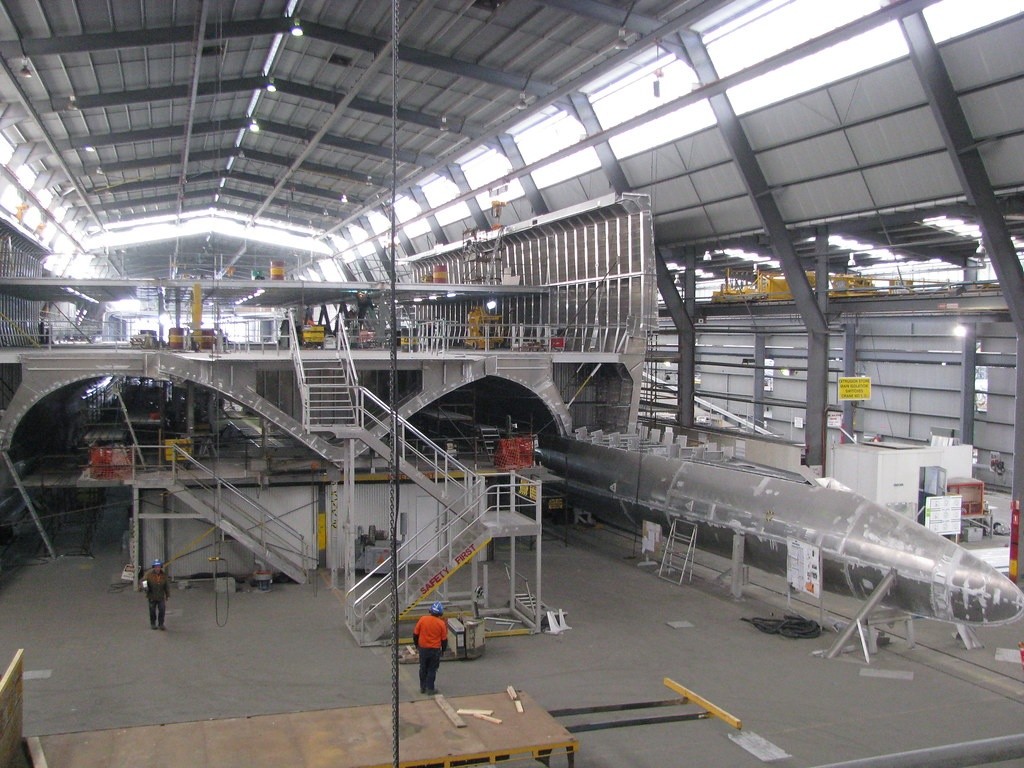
[413,600,448,695]
[141,559,170,631]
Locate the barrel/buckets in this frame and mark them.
[270,260,284,280]
[169,329,183,349]
[256,574,271,590]
[433,265,448,284]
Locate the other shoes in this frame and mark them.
[158,624,165,631]
[420,688,438,695]
[150,623,159,630]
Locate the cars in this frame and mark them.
[167,409,244,441]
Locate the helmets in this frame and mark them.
[152,559,163,568]
[431,601,443,615]
